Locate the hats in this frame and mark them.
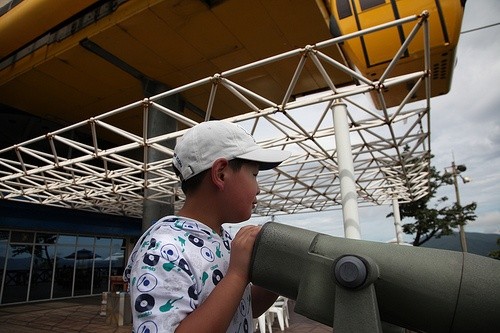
[171,121,292,181]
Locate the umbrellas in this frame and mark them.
[64,248,102,264]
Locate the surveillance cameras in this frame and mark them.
[463,177,470,183]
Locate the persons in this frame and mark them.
[122,121,292,333]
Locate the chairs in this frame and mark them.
[256,296,290,333]
